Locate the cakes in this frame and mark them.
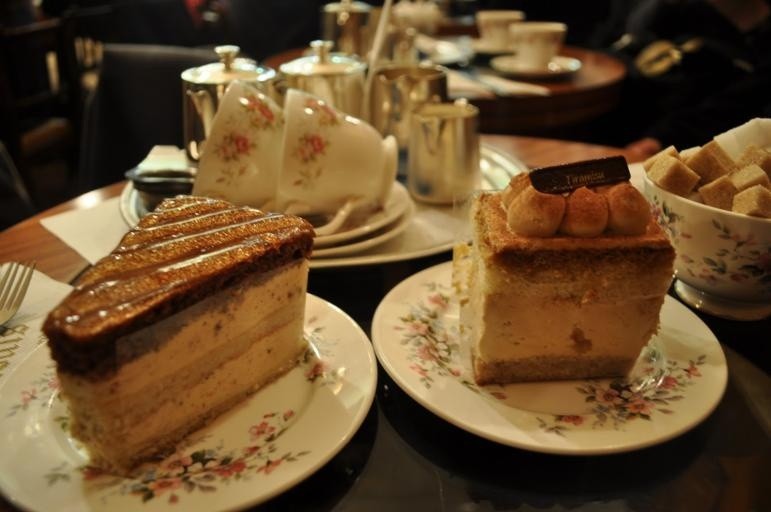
[39,192,317,477]
[458,152,680,388]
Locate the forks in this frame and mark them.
[0,244,39,330]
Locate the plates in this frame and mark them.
[117,139,528,272]
[368,255,727,451]
[490,55,581,79]
[312,181,413,262]
[2,288,378,512]
[470,41,514,56]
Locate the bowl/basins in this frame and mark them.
[642,162,770,325]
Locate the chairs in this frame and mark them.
[61,43,241,195]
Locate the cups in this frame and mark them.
[180,46,276,168]
[320,2,374,63]
[511,24,566,68]
[278,41,369,130]
[407,101,484,204]
[474,9,520,45]
[185,82,403,236]
[367,68,448,178]
